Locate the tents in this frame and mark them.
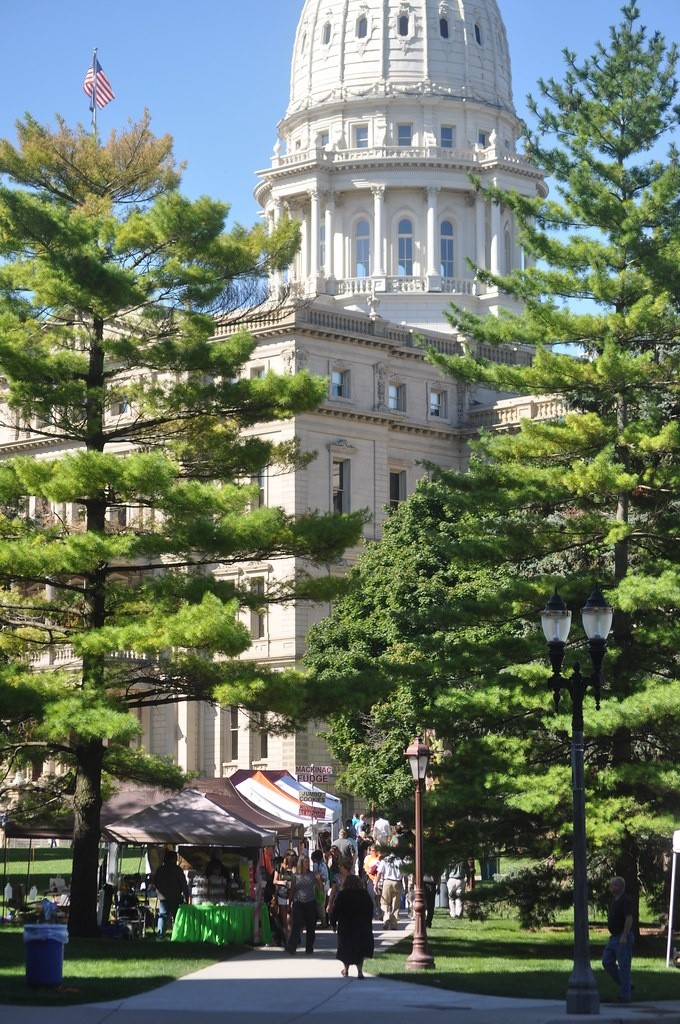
[4,767,344,939]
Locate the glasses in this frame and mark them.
[288,854,297,857]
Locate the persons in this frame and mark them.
[120,872,156,937]
[262,810,442,955]
[601,876,636,1003]
[329,873,375,979]
[444,855,470,920]
[8,897,61,914]
[153,851,190,940]
[202,858,231,900]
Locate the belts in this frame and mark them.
[452,877,465,879]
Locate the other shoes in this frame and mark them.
[286,946,296,954]
[306,949,313,953]
[341,970,348,977]
[358,975,364,979]
[383,918,390,930]
[450,914,463,920]
[391,926,396,930]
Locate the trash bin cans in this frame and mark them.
[434,879,449,909]
[23,923,67,986]
[478,856,500,880]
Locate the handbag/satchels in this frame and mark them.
[315,886,324,905]
[270,895,278,917]
[49,875,65,893]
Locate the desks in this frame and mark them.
[170,901,274,946]
[44,890,71,906]
[111,906,152,937]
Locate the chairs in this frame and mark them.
[117,908,143,939]
